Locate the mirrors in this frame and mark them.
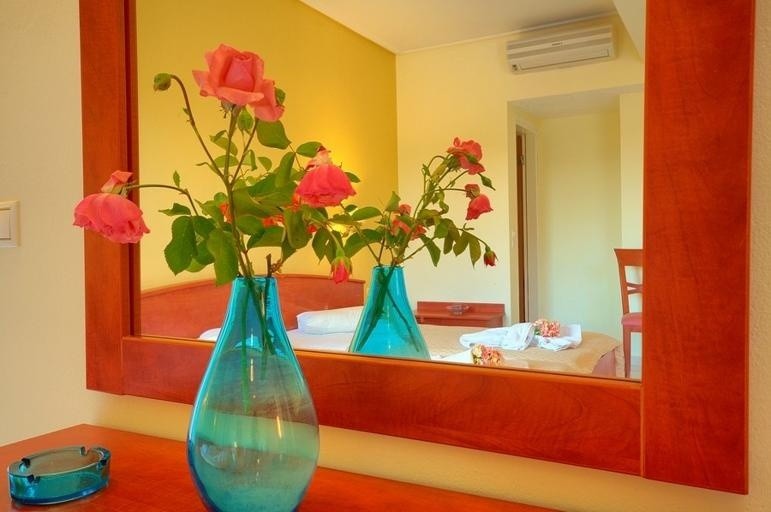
[78,1,754,495]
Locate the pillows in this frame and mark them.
[297,307,363,333]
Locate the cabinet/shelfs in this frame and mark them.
[413,301,505,327]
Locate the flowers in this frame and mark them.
[472,345,504,367]
[332,137,499,352]
[534,318,561,336]
[74,44,358,415]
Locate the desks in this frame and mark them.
[0,424,563,511]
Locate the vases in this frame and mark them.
[349,265,432,360]
[187,280,320,511]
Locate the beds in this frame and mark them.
[141,274,623,377]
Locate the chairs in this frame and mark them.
[613,249,643,378]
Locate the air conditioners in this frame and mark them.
[506,25,616,74]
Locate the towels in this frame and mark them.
[460,322,534,349]
[534,323,582,351]
[431,346,475,363]
